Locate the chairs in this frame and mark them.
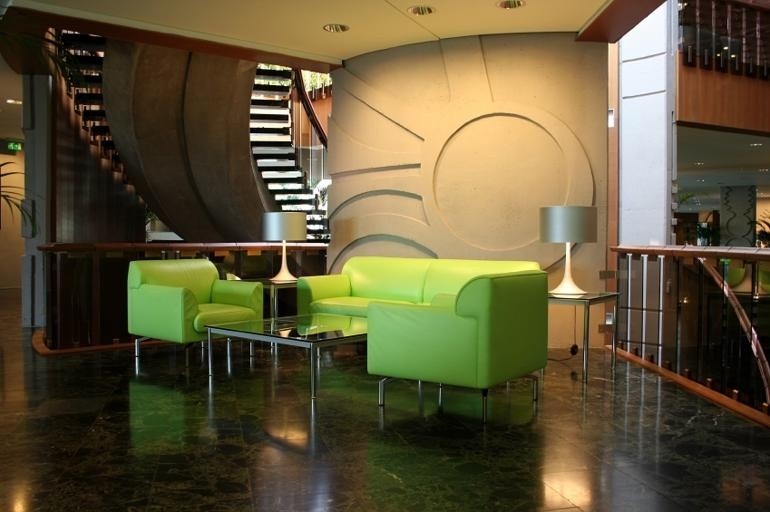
[366,271,550,424]
[128,257,264,371]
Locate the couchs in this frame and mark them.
[297,256,541,318]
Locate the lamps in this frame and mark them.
[261,212,307,283]
[540,206,598,290]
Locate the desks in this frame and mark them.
[550,291,619,385]
[263,280,297,318]
